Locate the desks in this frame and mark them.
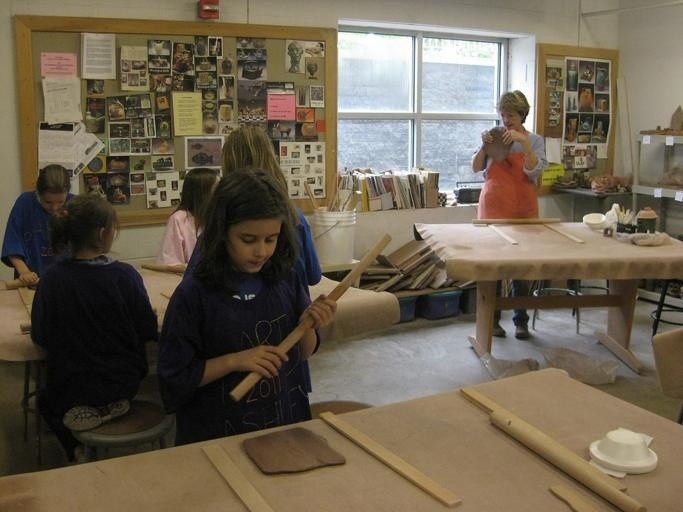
[0,266,400,466]
[0,367,682,511]
[415,222,683,376]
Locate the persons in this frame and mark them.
[30,192,157,466]
[154,167,339,447]
[221,125,324,302]
[287,40,303,75]
[162,168,222,275]
[305,43,325,58]
[471,91,547,340]
[1,164,80,288]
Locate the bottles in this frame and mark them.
[637,206,658,233]
[605,202,620,231]
[567,64,577,90]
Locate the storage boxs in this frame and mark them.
[387,290,462,323]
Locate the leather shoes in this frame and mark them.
[515,323,529,338]
[493,322,505,336]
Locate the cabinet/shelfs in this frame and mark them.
[632,132,682,234]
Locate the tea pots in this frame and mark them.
[253,50,263,59]
[241,61,263,80]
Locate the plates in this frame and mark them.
[553,182,578,188]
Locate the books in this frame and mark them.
[335,167,439,213]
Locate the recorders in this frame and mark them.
[456,181,485,202]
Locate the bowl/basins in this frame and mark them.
[582,212,605,228]
[588,427,660,476]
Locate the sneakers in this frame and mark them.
[64,400,130,432]
[72,444,105,465]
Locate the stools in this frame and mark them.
[531,279,582,333]
[310,401,374,423]
[73,401,177,464]
[650,279,683,339]
